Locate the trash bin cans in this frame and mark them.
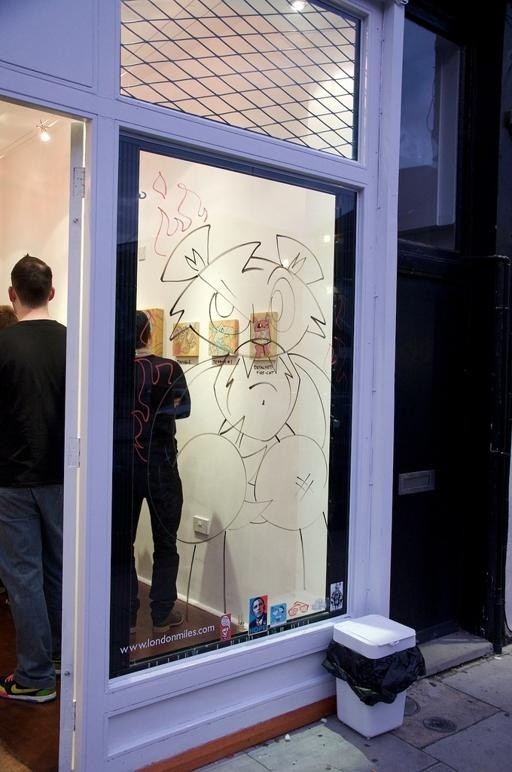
[332,617,417,740]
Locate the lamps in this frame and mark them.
[35,120,50,142]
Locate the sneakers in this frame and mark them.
[0,672,57,704]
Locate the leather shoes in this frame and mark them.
[152,610,184,634]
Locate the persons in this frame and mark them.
[249,598,268,634]
[120,308,200,637]
[328,581,343,610]
[1,251,69,706]
